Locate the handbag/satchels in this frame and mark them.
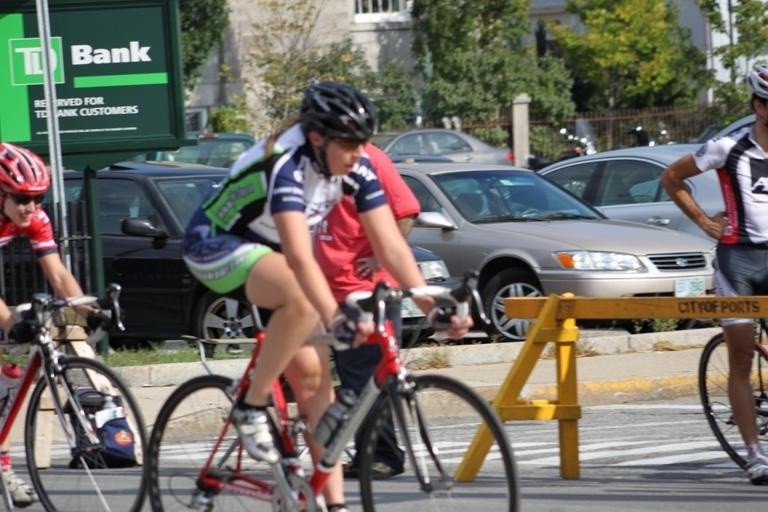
[61,390,143,469]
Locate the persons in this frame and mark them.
[313,142,421,479]
[181,82,474,512]
[660,63,768,485]
[0,141,125,508]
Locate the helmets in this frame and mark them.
[300,81,376,140]
[0,143,51,197]
[748,65,768,98]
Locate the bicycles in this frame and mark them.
[144,282,518,512]
[697,285,767,467]
[0,279,148,511]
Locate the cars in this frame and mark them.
[0,160,457,356]
[481,143,729,237]
[389,162,717,342]
[363,129,514,171]
[141,133,258,173]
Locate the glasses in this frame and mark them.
[10,194,45,204]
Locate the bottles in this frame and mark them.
[313,388,357,445]
[102,396,117,409]
[0,362,22,432]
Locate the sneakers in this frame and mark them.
[231,406,280,464]
[371,461,404,479]
[343,462,357,476]
[746,455,768,483]
[0,468,39,506]
